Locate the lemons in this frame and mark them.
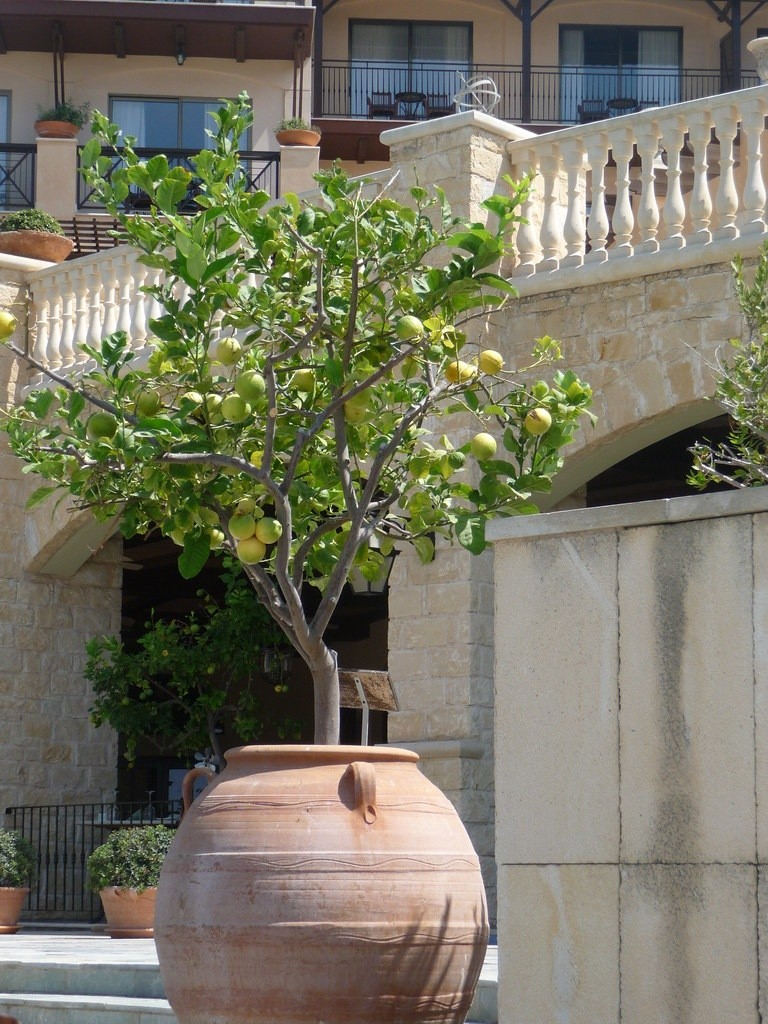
[236,371,265,401]
[0,312,17,340]
[341,379,372,423]
[471,432,497,462]
[525,408,552,434]
[89,410,117,441]
[181,392,252,425]
[228,499,283,565]
[171,524,225,549]
[395,315,423,344]
[479,349,503,376]
[138,389,161,414]
[217,337,242,366]
[294,369,316,392]
[446,360,474,384]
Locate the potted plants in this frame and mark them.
[273,115,321,145]
[0,86,600,1024]
[83,824,177,929]
[34,97,93,138]
[0,827,42,926]
[0,209,78,263]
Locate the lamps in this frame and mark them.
[175,42,186,66]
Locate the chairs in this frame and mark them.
[427,92,456,120]
[367,91,398,120]
[578,100,609,125]
[636,101,660,111]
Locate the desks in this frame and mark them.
[607,98,637,117]
[394,92,426,120]
